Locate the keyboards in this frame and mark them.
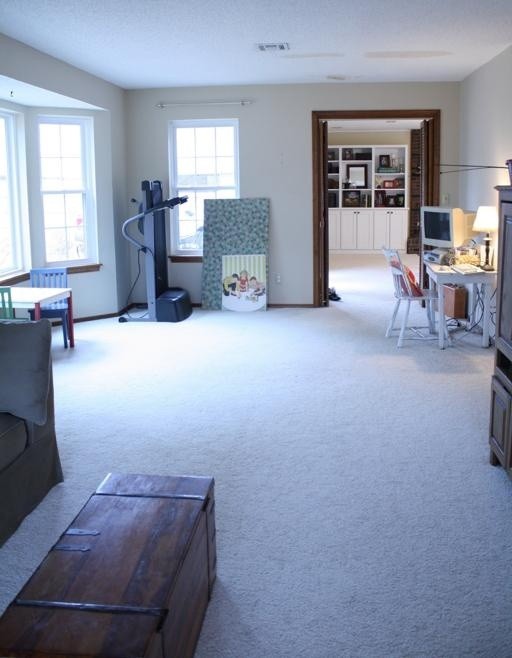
[451,264,485,276]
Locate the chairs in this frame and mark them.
[27,267,70,348]
[382,245,451,348]
[0,286,14,319]
[1,323,65,543]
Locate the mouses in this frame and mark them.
[440,266,447,270]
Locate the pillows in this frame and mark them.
[1,321,51,426]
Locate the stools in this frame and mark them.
[155,287,192,322]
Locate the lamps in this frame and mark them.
[472,206,498,271]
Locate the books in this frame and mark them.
[362,193,371,207]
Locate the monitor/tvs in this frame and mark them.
[495,202,512,363]
[419,206,478,251]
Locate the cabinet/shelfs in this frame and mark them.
[0,472,217,658]
[327,144,411,254]
[488,184,512,481]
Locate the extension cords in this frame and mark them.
[448,323,466,333]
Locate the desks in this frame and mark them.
[423,260,498,350]
[0,287,74,348]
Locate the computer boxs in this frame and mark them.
[430,248,450,265]
